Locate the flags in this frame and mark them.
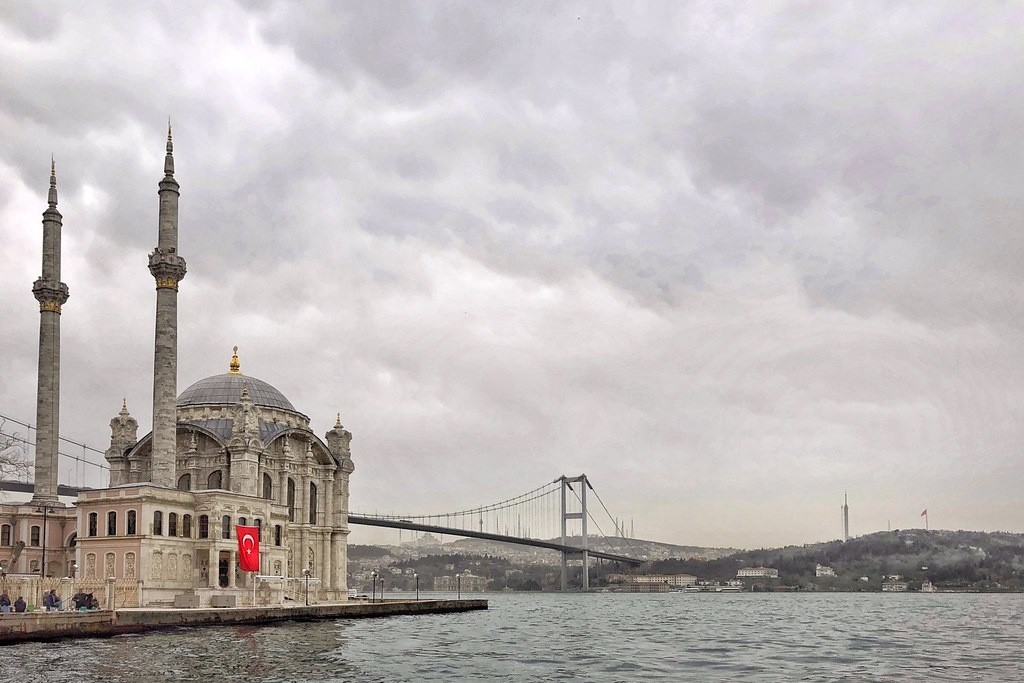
[236,525,259,572]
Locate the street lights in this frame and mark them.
[413,574,420,601]
[302,569,311,606]
[371,572,377,603]
[380,578,385,602]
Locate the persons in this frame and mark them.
[0,590,11,612]
[73,589,89,608]
[14,597,26,612]
[43,589,60,610]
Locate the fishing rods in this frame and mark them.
[52,576,125,612]
